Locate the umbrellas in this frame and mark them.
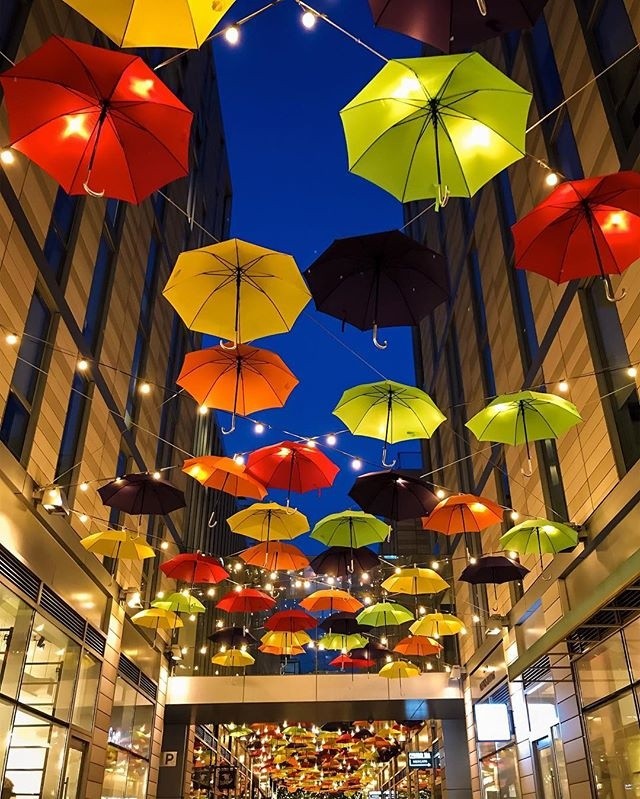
[421,492,504,561]
[498,517,579,581]
[331,380,448,467]
[302,230,451,350]
[176,341,300,435]
[244,440,341,516]
[181,456,269,528]
[380,562,451,620]
[458,556,531,611]
[339,51,533,213]
[0,34,194,208]
[79,526,466,697]
[463,389,585,477]
[96,471,186,540]
[227,720,426,797]
[161,237,313,351]
[367,0,549,55]
[511,171,640,302]
[62,0,237,51]
[348,469,441,540]
